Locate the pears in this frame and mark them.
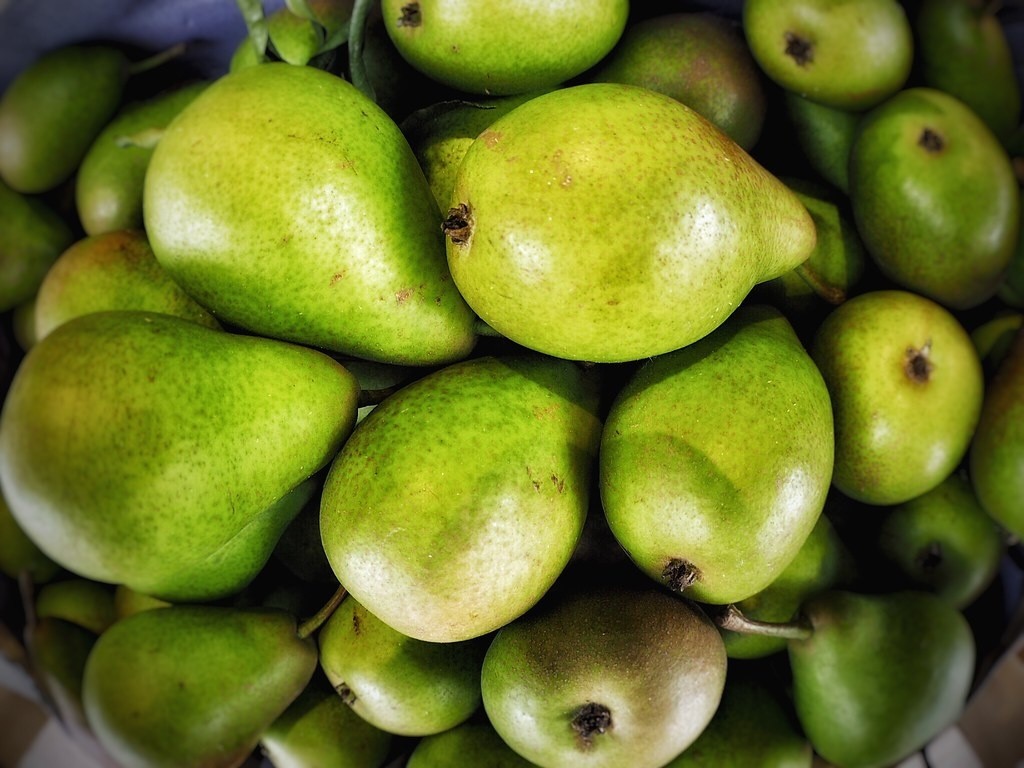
[1,1,1024,768]
